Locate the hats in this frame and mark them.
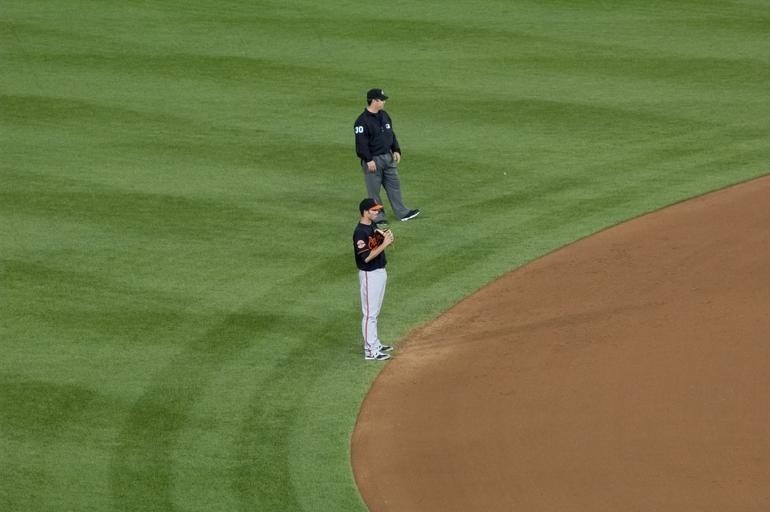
[359,198,383,211]
[366,87,389,101]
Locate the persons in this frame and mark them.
[353,88,421,227]
[351,197,396,362]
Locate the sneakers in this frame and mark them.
[365,345,394,360]
[400,208,421,222]
